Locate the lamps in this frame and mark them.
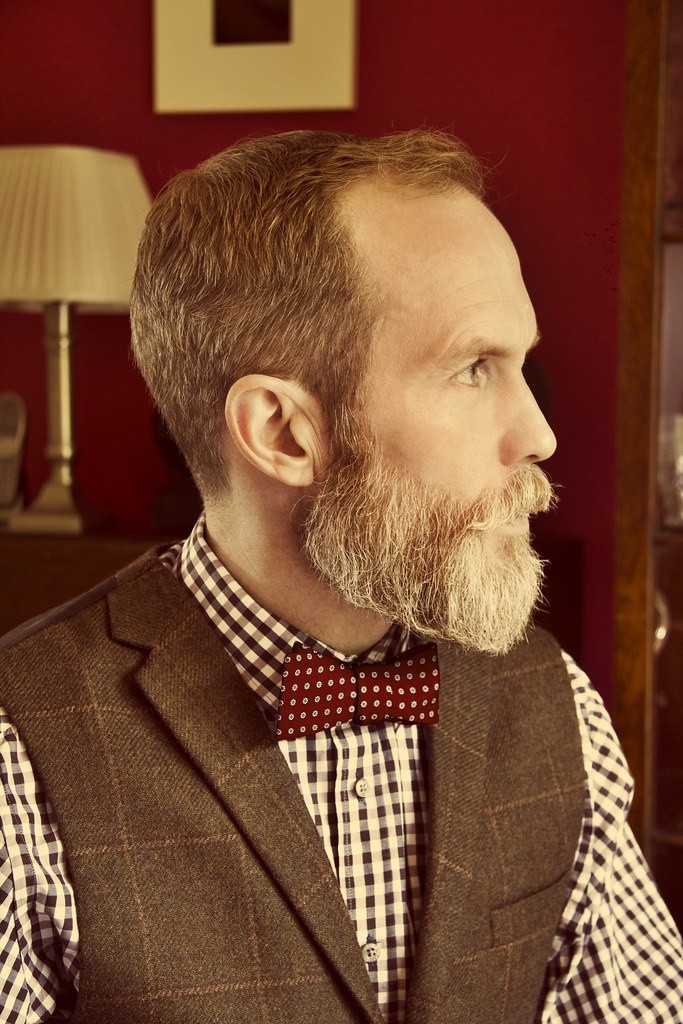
[0,145,152,534]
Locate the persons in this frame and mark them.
[0,123,683,1023]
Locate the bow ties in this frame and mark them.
[274,643,439,738]
